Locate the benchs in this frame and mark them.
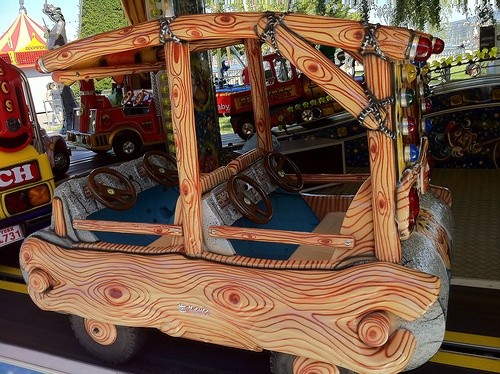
[288,212,347,260]
[148,234,175,248]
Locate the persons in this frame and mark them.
[45,82,62,124]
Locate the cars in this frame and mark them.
[18,12,454,374]
[65,75,166,159]
[215,51,322,141]
[0,55,61,250]
[29,119,72,174]
